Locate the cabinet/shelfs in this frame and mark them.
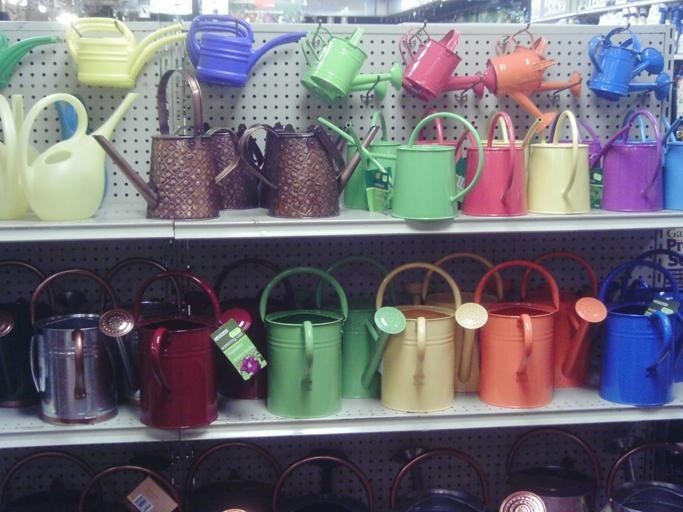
[140,1,681,33]
[0,19,681,512]
